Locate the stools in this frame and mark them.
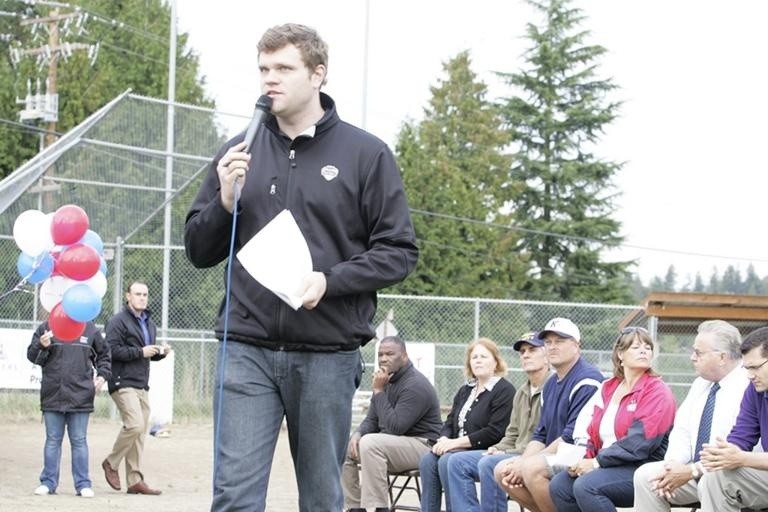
[386,468,422,511]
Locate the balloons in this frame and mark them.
[13,203,108,342]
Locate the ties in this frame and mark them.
[695,383,720,462]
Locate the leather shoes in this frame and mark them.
[127,480,161,496]
[101,459,121,491]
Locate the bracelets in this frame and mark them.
[691,462,700,482]
[592,456,601,468]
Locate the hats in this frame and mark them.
[537,318,581,342]
[513,330,544,351]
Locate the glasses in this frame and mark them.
[740,360,768,371]
[617,327,649,343]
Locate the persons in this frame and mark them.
[491,317,605,512]
[341,334,441,510]
[101,280,172,496]
[632,316,750,512]
[418,337,516,512]
[446,330,555,512]
[25,318,113,496]
[696,326,768,512]
[547,326,676,512]
[181,19,420,511]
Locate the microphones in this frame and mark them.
[239,94,273,177]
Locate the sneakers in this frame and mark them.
[35,485,50,495]
[80,488,94,498]
